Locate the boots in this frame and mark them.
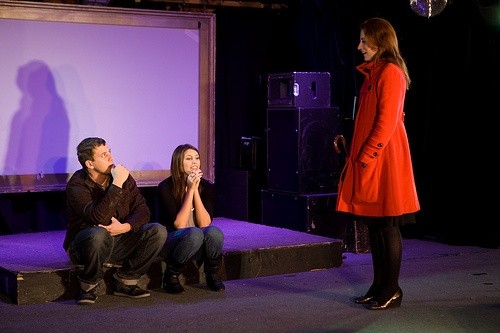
[204,255,225,291]
[164,258,188,295]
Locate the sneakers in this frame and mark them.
[77,287,98,303]
[114,282,150,299]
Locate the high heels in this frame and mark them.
[366,287,403,310]
[354,296,373,303]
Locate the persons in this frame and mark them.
[335,17,421,311]
[156,144,225,295]
[62,137,167,304]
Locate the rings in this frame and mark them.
[191,172,196,177]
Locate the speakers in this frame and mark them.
[267,71,341,194]
